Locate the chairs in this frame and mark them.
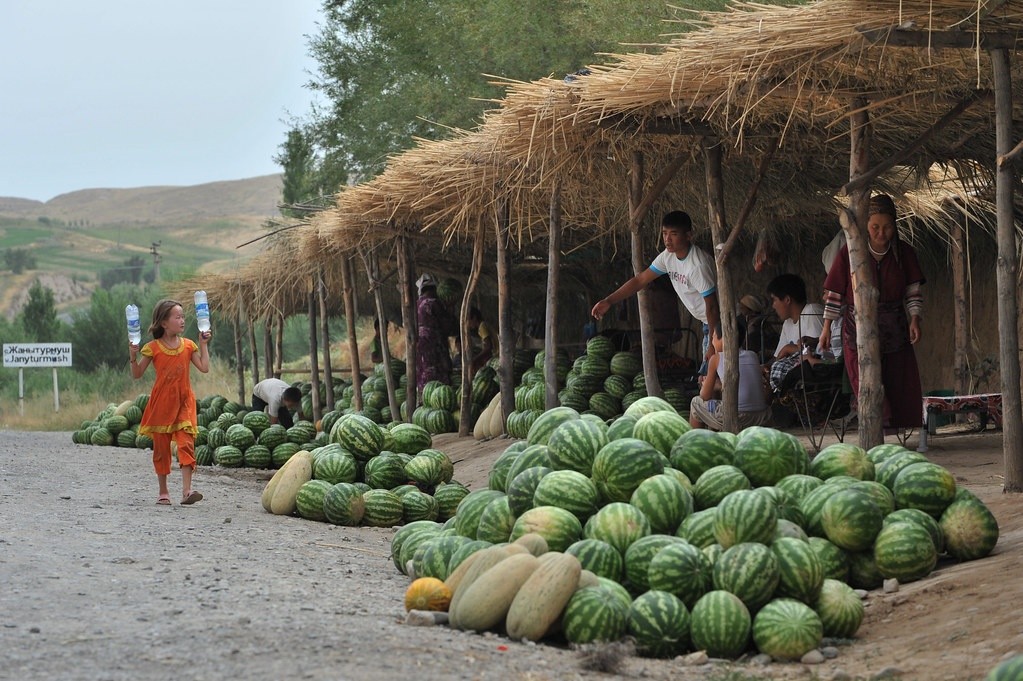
[758,312,916,452]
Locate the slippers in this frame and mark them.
[156,494,171,505]
[180,491,203,504]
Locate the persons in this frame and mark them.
[251,378,305,430]
[689,319,773,435]
[591,211,748,376]
[455,306,499,367]
[759,274,841,426]
[414,273,457,407]
[369,319,396,368]
[819,195,928,436]
[128,300,212,504]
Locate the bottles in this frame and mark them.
[125,304,142,346]
[194,290,210,333]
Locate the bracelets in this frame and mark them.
[129,356,136,363]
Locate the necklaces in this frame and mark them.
[868,237,891,256]
[161,338,181,355]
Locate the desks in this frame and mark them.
[917,393,1002,452]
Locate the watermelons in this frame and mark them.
[73,336,700,526]
[983,654,1023,681]
[392,394,999,663]
